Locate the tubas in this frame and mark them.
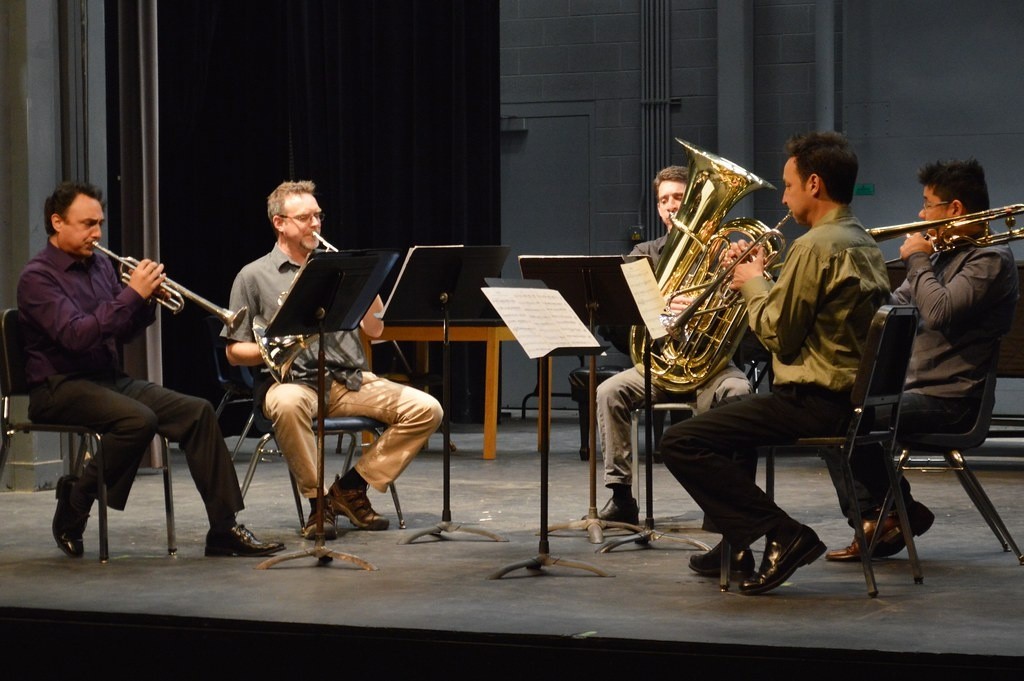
[628,134,783,394]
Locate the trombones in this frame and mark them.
[666,203,1024,320]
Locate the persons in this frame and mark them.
[660,135,890,594]
[596,165,750,530]
[219,180,445,538]
[826,156,1016,564]
[16,181,287,558]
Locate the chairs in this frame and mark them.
[630,359,776,537]
[0,308,178,564]
[707,300,927,595]
[870,337,1024,568]
[202,313,345,463]
[232,361,412,534]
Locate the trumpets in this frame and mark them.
[663,209,792,344]
[91,239,249,339]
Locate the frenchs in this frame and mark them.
[252,230,340,385]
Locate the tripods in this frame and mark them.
[255,243,713,580]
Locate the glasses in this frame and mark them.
[277,210,326,224]
[923,198,949,211]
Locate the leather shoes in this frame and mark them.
[739,523,827,594]
[826,499,936,562]
[51,475,87,559]
[203,523,279,556]
[688,542,756,578]
[582,499,640,525]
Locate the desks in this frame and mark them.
[352,317,553,462]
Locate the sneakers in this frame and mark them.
[305,511,337,538]
[330,479,390,531]
